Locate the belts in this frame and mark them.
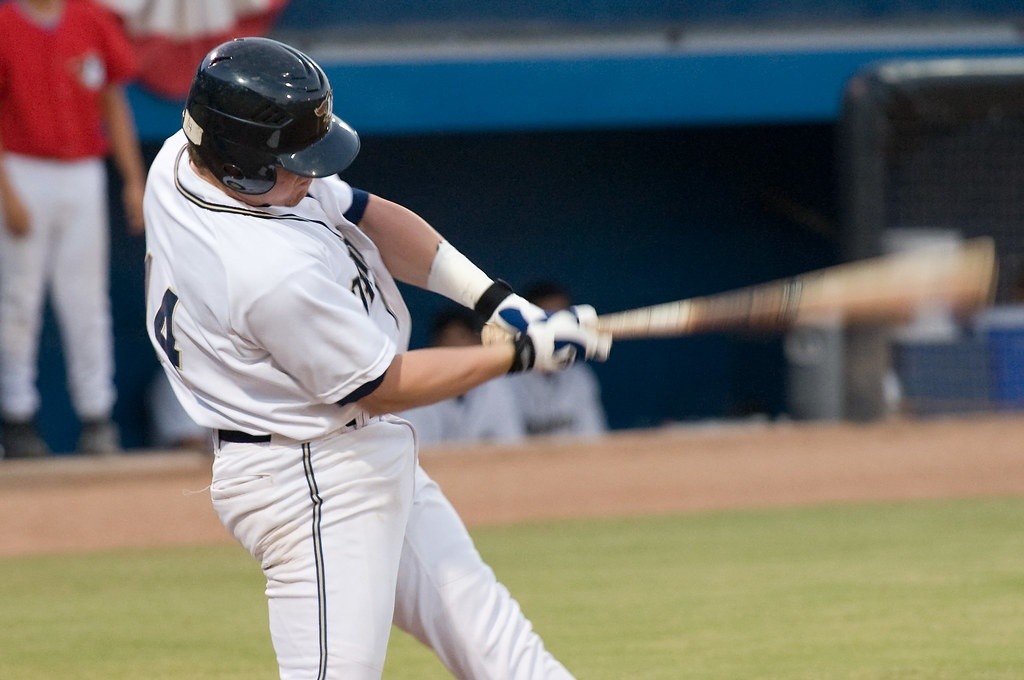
[218,419,356,443]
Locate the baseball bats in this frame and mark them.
[480,239,1002,348]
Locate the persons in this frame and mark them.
[143,38,611,680]
[0,1,204,458]
[393,280,604,453]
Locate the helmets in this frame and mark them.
[182,37,361,195]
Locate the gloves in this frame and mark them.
[510,303,613,375]
[472,277,551,342]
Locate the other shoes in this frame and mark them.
[0,422,53,460]
[75,417,125,455]
